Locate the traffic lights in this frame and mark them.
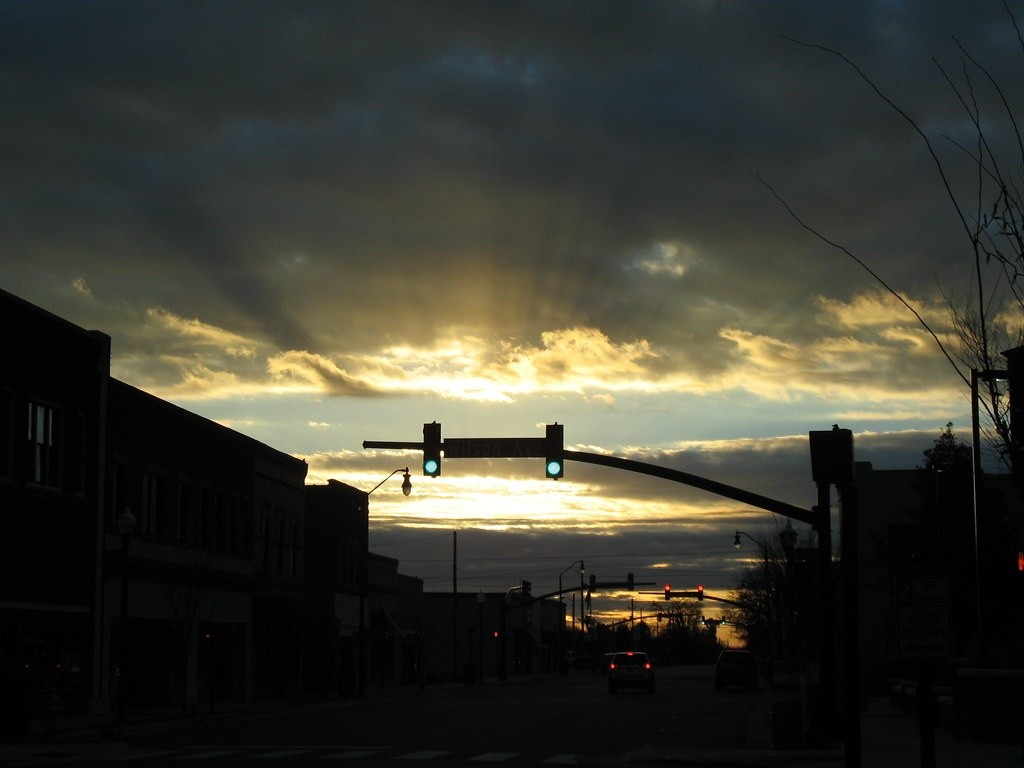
[697,585,703,601]
[665,585,671,600]
[423,422,440,477]
[546,425,564,479]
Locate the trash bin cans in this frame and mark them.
[953,667,1024,743]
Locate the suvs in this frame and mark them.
[601,653,615,675]
[608,652,656,695]
[714,650,759,694]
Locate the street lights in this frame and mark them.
[733,531,770,660]
[359,467,411,699]
[558,560,586,675]
